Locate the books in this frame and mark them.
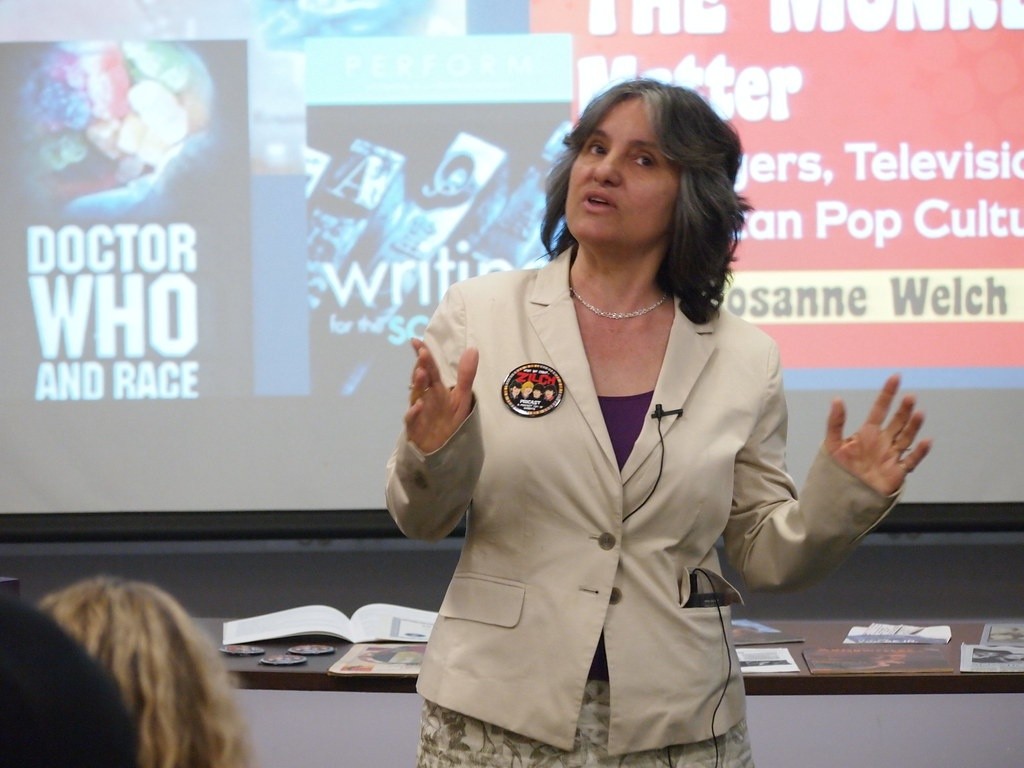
[216,602,442,648]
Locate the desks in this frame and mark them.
[185,619,1024,768]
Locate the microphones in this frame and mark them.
[656,404,662,421]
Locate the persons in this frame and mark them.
[35,574,256,768]
[0,602,136,768]
[383,72,930,768]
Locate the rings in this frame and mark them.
[408,383,431,391]
[893,444,905,452]
[898,460,914,473]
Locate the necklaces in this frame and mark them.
[568,288,671,320]
